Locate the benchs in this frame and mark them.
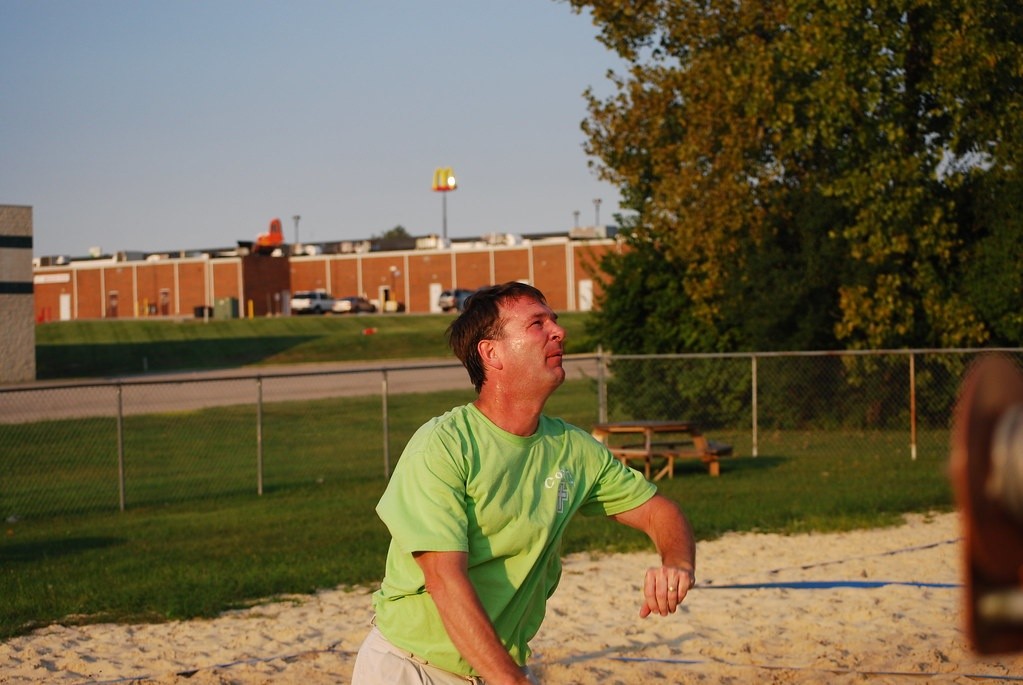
[606,441,734,482]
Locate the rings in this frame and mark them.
[668,586,676,592]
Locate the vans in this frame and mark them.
[289,290,333,314]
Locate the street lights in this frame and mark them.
[594,198,601,224]
[573,210,580,231]
[293,215,302,248]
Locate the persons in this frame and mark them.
[351,282,695,685]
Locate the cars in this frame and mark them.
[330,297,378,314]
[439,290,473,311]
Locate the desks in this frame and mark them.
[589,421,721,483]
